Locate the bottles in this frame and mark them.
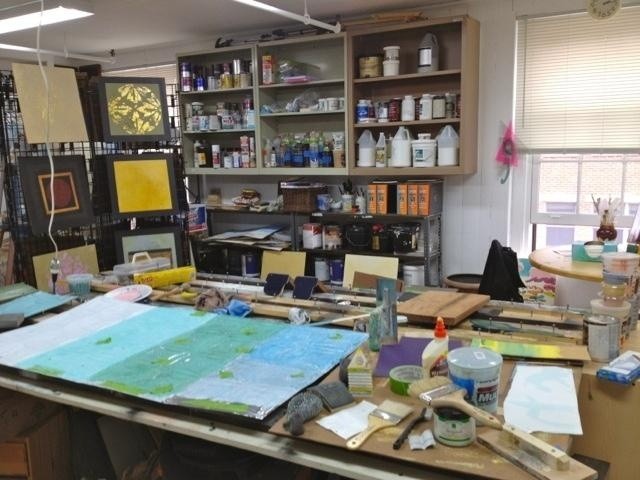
[354,92,464,123]
[262,129,332,168]
[193,137,213,168]
[376,129,387,168]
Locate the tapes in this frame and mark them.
[389,365,426,396]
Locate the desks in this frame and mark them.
[0,279,640,480]
[528,244,640,310]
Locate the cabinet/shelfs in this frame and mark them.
[175,41,257,177]
[253,30,348,174]
[187,205,441,287]
[348,16,479,177]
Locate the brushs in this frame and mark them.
[346,398,414,452]
[406,376,503,432]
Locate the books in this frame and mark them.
[203,224,290,251]
[366,179,443,215]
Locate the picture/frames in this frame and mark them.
[17,76,183,293]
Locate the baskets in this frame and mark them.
[281,182,327,214]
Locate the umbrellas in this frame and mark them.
[494,118,520,183]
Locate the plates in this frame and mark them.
[103,284,153,303]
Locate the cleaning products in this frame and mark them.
[422,318,450,379]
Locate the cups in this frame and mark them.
[317,194,329,211]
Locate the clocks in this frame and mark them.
[587,0,621,20]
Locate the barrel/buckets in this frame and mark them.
[583,252,640,361]
[356,125,459,168]
[448,346,503,415]
